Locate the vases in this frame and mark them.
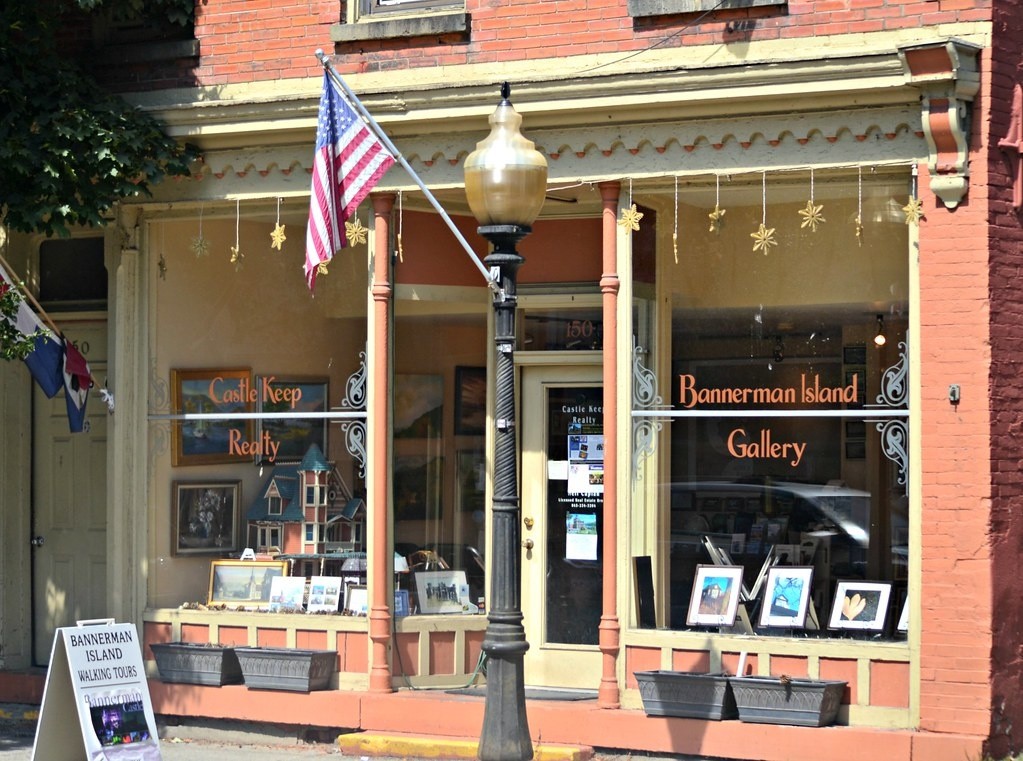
[149,642,250,687]
[728,675,849,727]
[633,670,748,721]
[234,646,338,692]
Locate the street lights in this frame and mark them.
[462,79,549,761]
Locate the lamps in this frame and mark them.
[874,314,887,346]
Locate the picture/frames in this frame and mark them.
[172,479,241,558]
[757,566,815,629]
[412,569,472,614]
[394,590,410,616]
[207,560,291,611]
[826,580,893,632]
[255,374,330,466]
[170,367,255,467]
[345,584,368,616]
[454,366,485,436]
[686,564,744,627]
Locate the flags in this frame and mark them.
[0,264,91,434]
[304,66,396,289]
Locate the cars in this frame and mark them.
[659,478,909,586]
